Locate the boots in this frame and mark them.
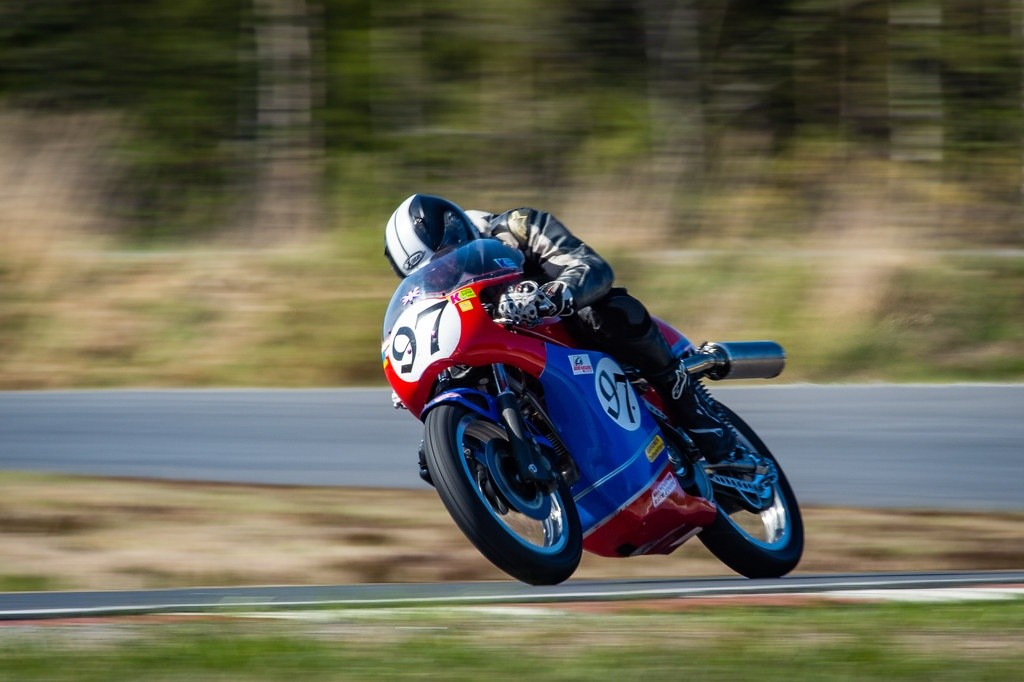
[648,358,736,465]
[420,448,509,516]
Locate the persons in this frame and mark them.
[382,194,737,515]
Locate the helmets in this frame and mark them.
[383,193,484,293]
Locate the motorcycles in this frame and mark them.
[380,236,803,586]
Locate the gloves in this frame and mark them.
[498,281,574,328]
